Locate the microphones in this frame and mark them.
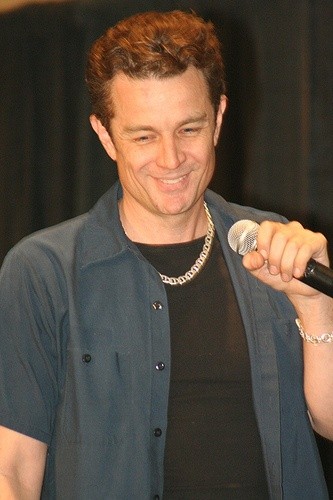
[226,219,333,299]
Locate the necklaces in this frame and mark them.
[158,199,215,285]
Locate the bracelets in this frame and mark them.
[294,318,332,345]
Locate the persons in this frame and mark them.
[0,9,332,500]
